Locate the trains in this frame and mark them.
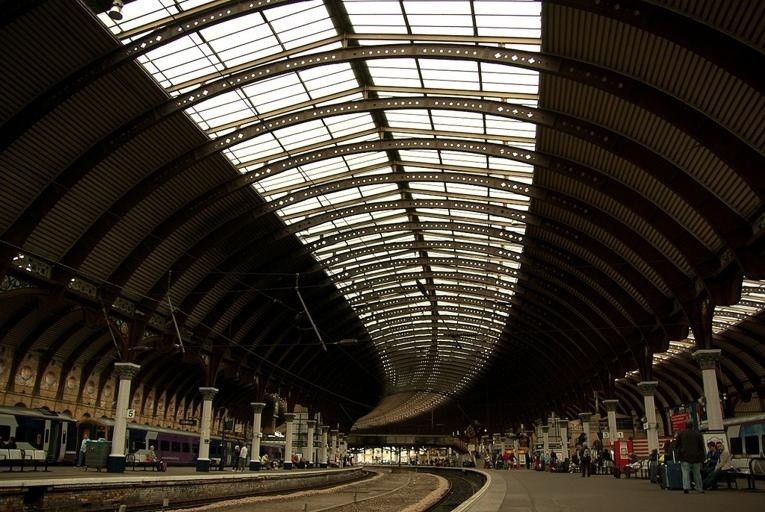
[701,414,765,474]
[0,405,284,464]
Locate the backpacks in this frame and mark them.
[583,449,590,458]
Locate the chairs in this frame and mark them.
[636,459,652,479]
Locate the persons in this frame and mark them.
[231,444,352,472]
[475,420,732,493]
[0,437,17,449]
[150,444,162,472]
[77,433,91,467]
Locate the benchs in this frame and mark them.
[210,457,222,470]
[727,457,765,490]
[126,454,160,471]
[0,448,48,471]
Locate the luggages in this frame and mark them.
[660,463,683,490]
[650,455,659,484]
